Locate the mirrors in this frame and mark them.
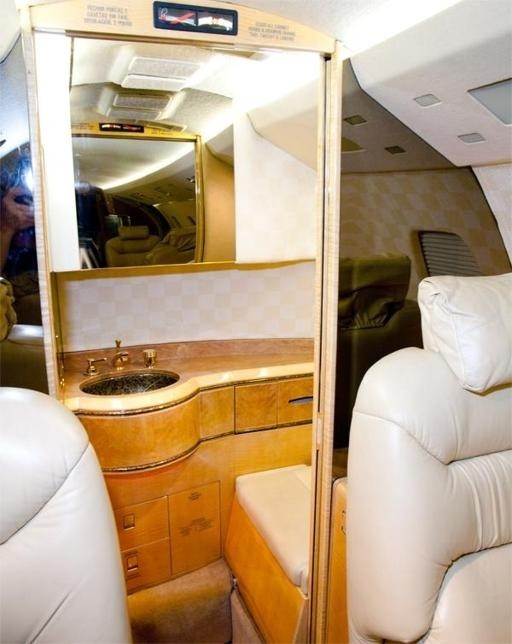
[71,122,205,263]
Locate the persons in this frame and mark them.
[0,139,37,277]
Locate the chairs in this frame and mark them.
[106,227,197,267]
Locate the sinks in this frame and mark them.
[78,365,181,398]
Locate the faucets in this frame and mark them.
[111,351,131,369]
